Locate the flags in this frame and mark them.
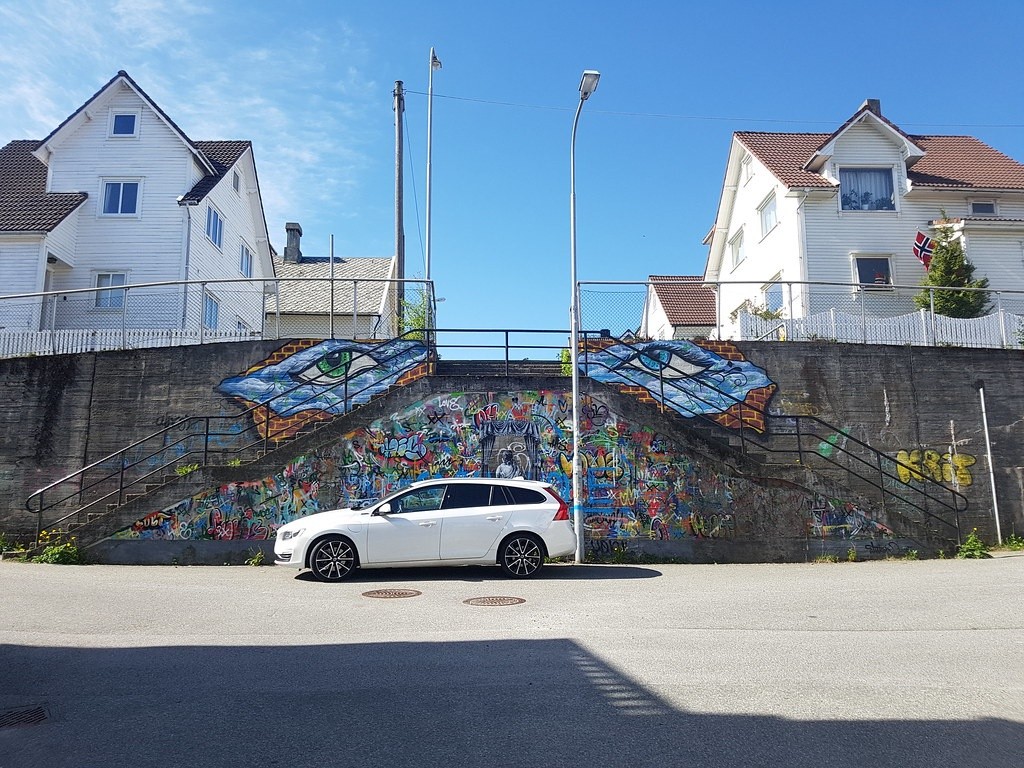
[913,226,939,271]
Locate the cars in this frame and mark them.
[274,475,578,577]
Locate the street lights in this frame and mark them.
[567,69,601,568]
[424,47,446,335]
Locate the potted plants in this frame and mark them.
[841,189,858,210]
[874,197,895,210]
[861,191,873,210]
